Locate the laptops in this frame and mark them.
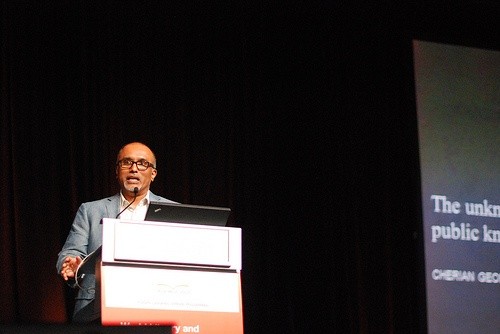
[144,201,231,227]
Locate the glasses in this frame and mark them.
[118,158,156,171]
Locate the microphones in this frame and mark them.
[115,186,138,219]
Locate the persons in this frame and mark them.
[56,141,180,321]
